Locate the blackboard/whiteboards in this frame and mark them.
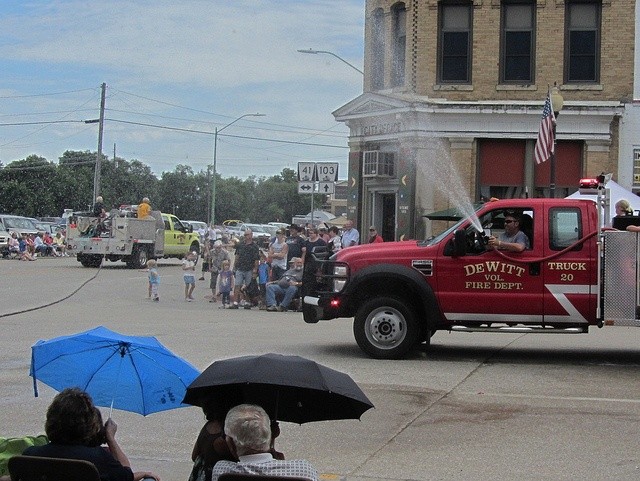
[67,211,201,269]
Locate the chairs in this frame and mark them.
[199,277,205,280]
[244,304,250,309]
[267,305,277,311]
[228,303,238,309]
[209,297,216,303]
[275,306,283,312]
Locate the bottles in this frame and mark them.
[289,257,297,263]
[369,225,376,231]
[317,224,328,231]
[287,224,301,232]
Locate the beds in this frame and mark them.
[421,198,513,222]
[29,325,201,419]
[179,353,375,426]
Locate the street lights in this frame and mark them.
[0,435,49,478]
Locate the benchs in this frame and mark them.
[535,83,557,166]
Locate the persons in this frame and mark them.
[327,226,341,251]
[210,403,319,481]
[182,252,197,302]
[253,254,270,305]
[187,399,239,481]
[217,260,235,309]
[22,387,161,481]
[199,240,212,280]
[229,230,259,310]
[209,240,228,302]
[267,228,285,280]
[342,220,360,248]
[487,215,531,253]
[368,226,384,243]
[285,224,301,268]
[140,259,160,302]
[138,197,153,219]
[266,257,304,312]
[9,231,70,261]
[615,200,634,216]
[197,224,234,243]
[94,196,106,218]
[301,222,327,263]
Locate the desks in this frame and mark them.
[295,48,363,84]
[211,114,263,229]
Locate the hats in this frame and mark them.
[0,222,12,257]
[0,215,66,246]
[180,221,207,234]
[223,222,292,246]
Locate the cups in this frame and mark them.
[276,231,282,234]
[504,219,518,224]
[244,233,250,236]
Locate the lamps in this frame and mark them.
[6,455,101,480]
[218,473,313,480]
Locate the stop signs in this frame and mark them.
[300,191,640,359]
